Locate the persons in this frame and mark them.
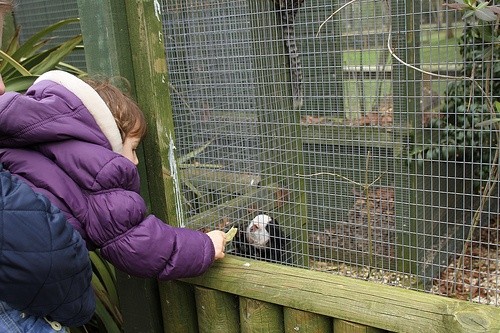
[0,70,229,333]
